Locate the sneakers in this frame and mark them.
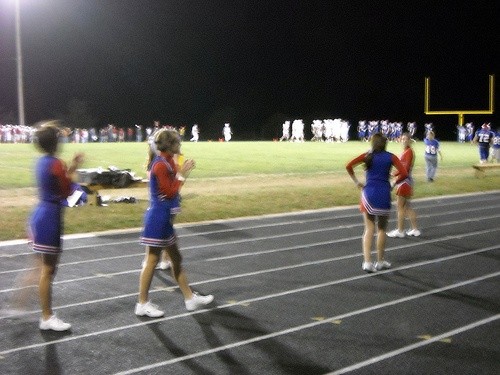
[39,312,72,332]
[184,291,214,311]
[134,300,165,318]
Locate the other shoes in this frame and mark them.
[375,260,392,270]
[160,260,172,270]
[386,229,405,238]
[406,229,421,236]
[140,259,162,269]
[363,262,377,273]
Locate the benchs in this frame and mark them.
[472,163,500,179]
[77,179,149,207]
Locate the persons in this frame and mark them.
[385,133,421,238]
[346,133,408,274]
[133,129,214,317]
[222,123,233,142]
[0,124,200,142]
[279,118,500,182]
[0,125,85,331]
